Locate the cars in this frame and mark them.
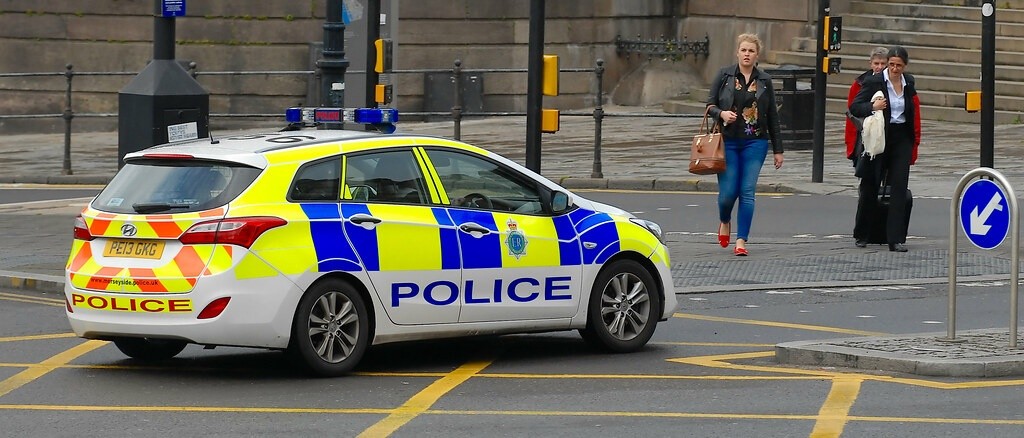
[63,108,677,379]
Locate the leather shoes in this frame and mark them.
[854,238,867,247]
[888,242,908,252]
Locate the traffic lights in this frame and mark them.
[375,38,393,74]
[375,84,393,104]
[823,17,842,51]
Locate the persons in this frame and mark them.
[706,34,784,255]
[849,47,921,252]
[845,46,889,167]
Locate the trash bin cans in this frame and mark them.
[761,68,816,152]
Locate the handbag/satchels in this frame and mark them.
[689,105,727,175]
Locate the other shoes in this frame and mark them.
[717,221,731,248]
[733,247,749,256]
